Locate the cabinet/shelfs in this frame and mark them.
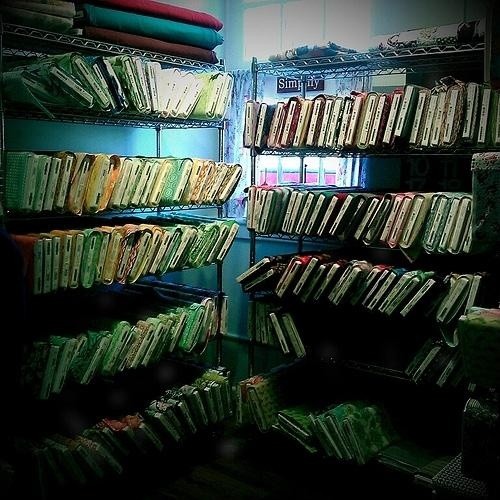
[246,22,500,500]
[0,19,225,500]
